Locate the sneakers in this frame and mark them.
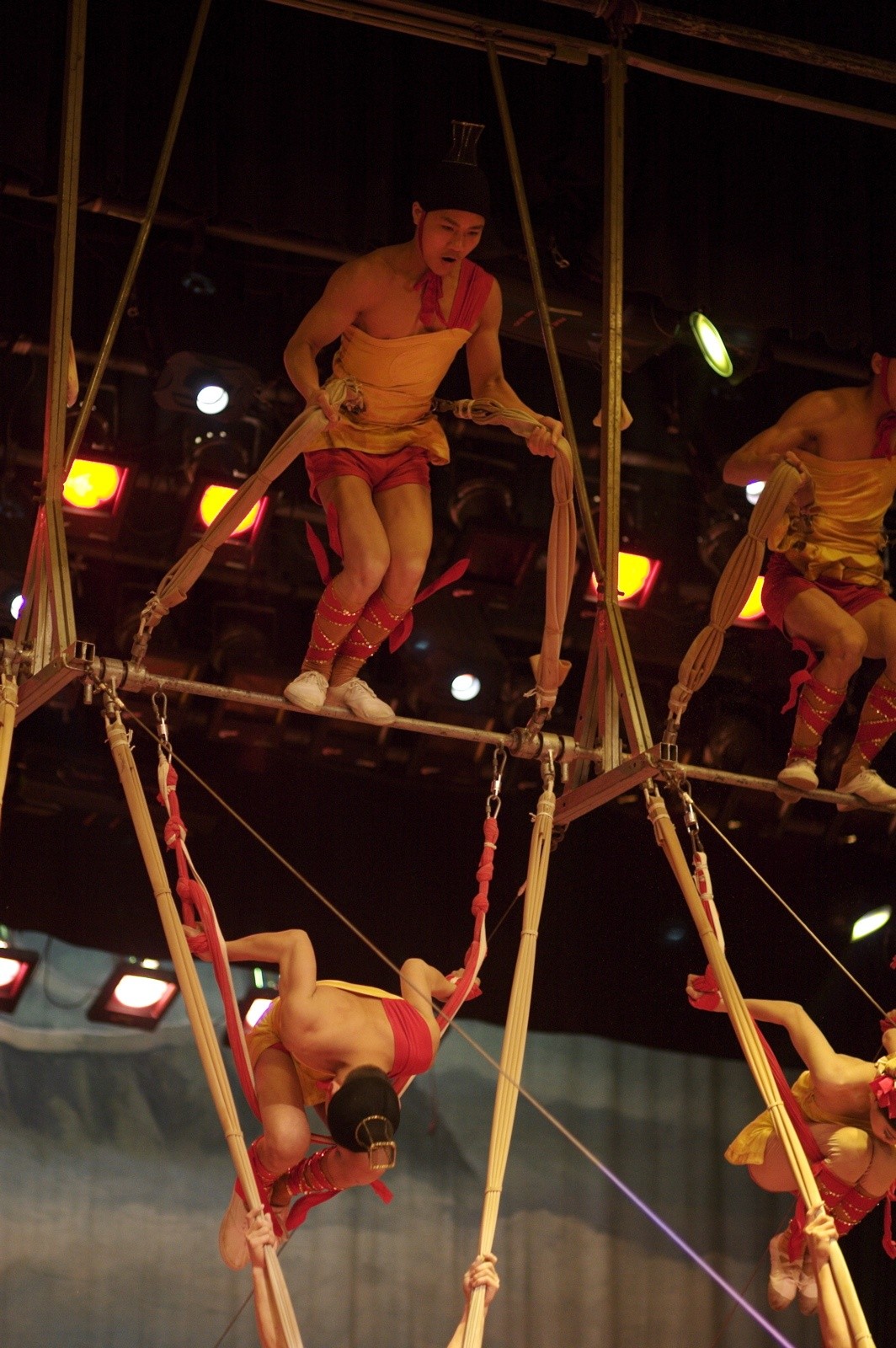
[835,767,896,812]
[798,1256,818,1314]
[271,1204,291,1248]
[220,1184,273,1269]
[329,679,396,726]
[285,667,330,713]
[768,1234,806,1311]
[776,761,818,804]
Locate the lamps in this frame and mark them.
[675,301,789,388]
[0,933,38,1014]
[447,663,481,702]
[6,588,24,620]
[113,604,203,730]
[192,366,229,415]
[60,429,130,519]
[573,512,664,611]
[206,621,294,749]
[449,481,546,595]
[172,448,270,566]
[810,886,892,944]
[85,937,179,1031]
[728,481,769,505]
[696,516,775,630]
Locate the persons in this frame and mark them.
[688,963,896,1314]
[445,1252,500,1348]
[723,323,896,810]
[189,925,482,1271]
[281,119,562,726]
